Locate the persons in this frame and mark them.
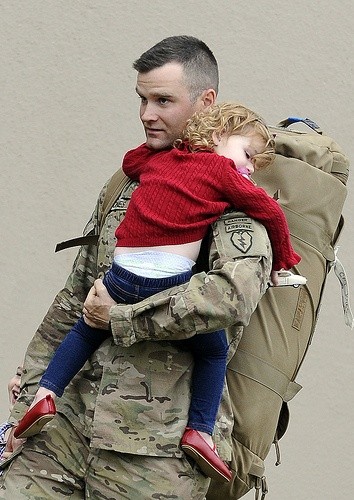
[0,35,354,500]
[15,101,301,485]
[0,363,28,477]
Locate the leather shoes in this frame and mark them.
[179,429,233,485]
[12,395,57,440]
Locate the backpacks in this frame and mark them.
[208,116,352,499]
